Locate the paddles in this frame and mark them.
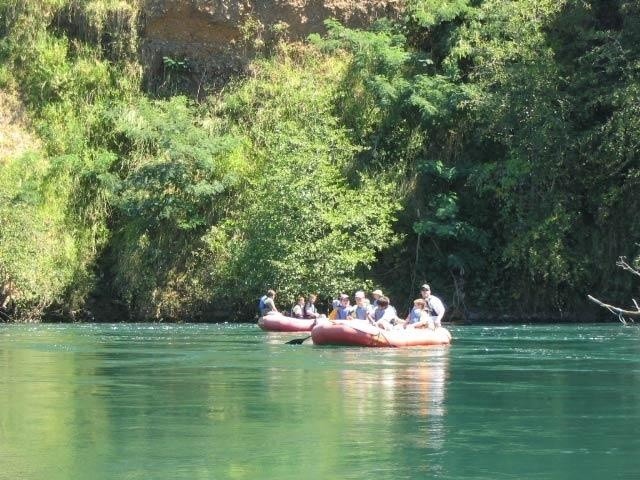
[285,335,314,345]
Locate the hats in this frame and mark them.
[355,291,365,298]
[421,284,430,291]
[372,290,383,296]
[340,294,349,299]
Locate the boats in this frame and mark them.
[258,313,451,347]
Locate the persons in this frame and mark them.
[354,291,372,320]
[369,296,398,327]
[420,283,446,327]
[305,294,320,318]
[403,298,434,329]
[291,296,305,318]
[334,294,354,319]
[373,289,384,306]
[258,290,279,315]
